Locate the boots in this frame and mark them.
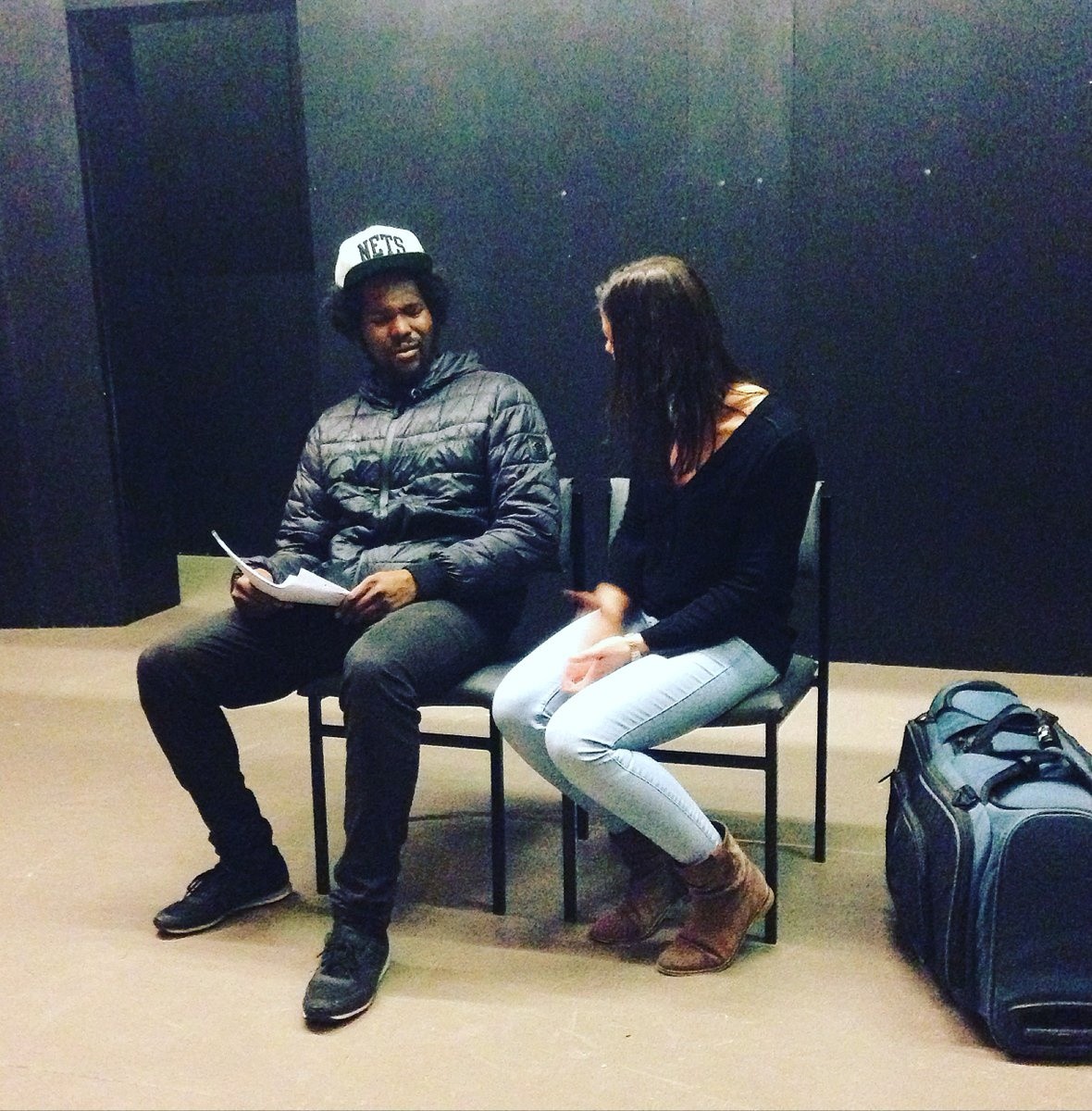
[589,825,691,944]
[656,820,775,976]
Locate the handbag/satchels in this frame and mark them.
[886,680,1092,1061]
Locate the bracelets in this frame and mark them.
[624,633,642,661]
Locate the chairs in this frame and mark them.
[306,476,591,918]
[575,478,827,938]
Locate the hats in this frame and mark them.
[334,225,436,290]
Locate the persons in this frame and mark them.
[493,255,822,977]
[137,224,564,1024]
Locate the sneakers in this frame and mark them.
[302,929,392,1022]
[152,844,292,933]
